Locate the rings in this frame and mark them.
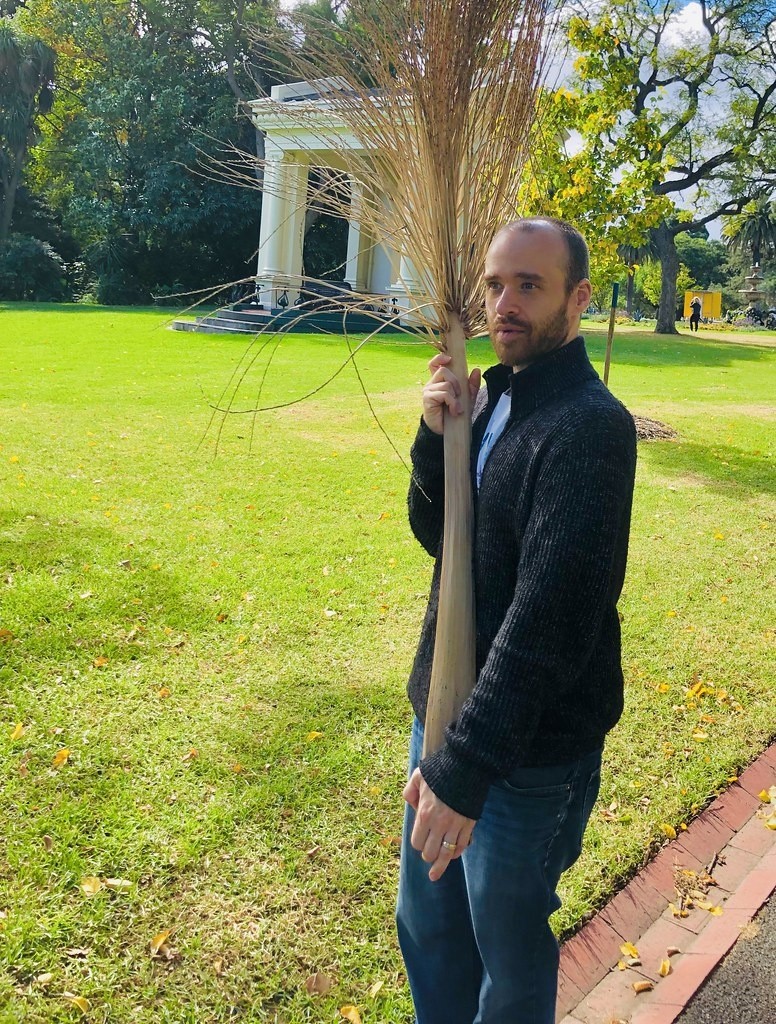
[442,841,456,850]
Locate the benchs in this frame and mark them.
[304,280,369,310]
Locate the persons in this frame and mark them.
[395,216,637,1024]
[690,297,701,331]
